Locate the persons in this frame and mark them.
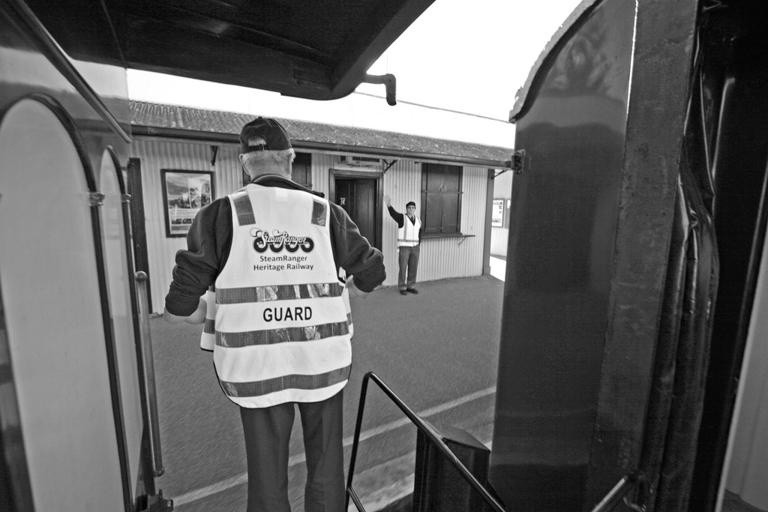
[165,114,387,512]
[384,193,426,295]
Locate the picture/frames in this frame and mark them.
[159,167,217,238]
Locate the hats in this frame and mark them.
[239,116,292,154]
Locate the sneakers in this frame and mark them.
[407,288,419,294]
[400,290,408,294]
